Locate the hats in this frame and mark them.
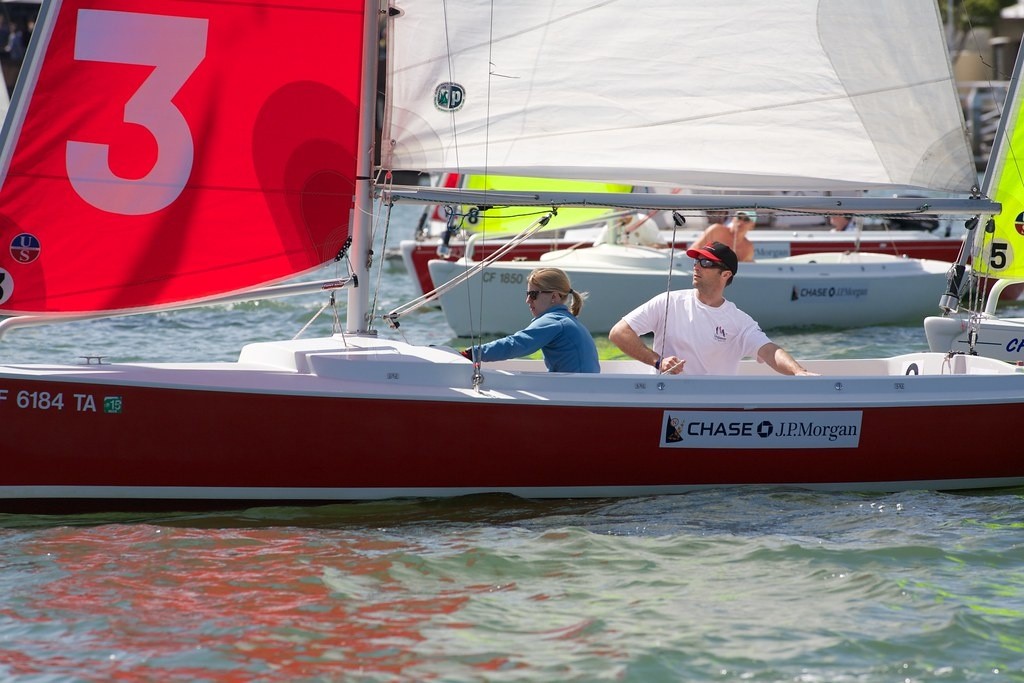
[686,242,738,273]
[735,210,757,222]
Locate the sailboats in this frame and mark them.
[0,1,1024,522]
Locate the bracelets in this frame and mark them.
[655,356,664,369]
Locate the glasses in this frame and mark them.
[737,216,749,222]
[527,290,562,300]
[693,258,735,275]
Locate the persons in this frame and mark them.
[689,210,757,262]
[592,207,669,247]
[608,241,821,376]
[828,216,858,231]
[459,268,601,373]
[706,211,732,229]
